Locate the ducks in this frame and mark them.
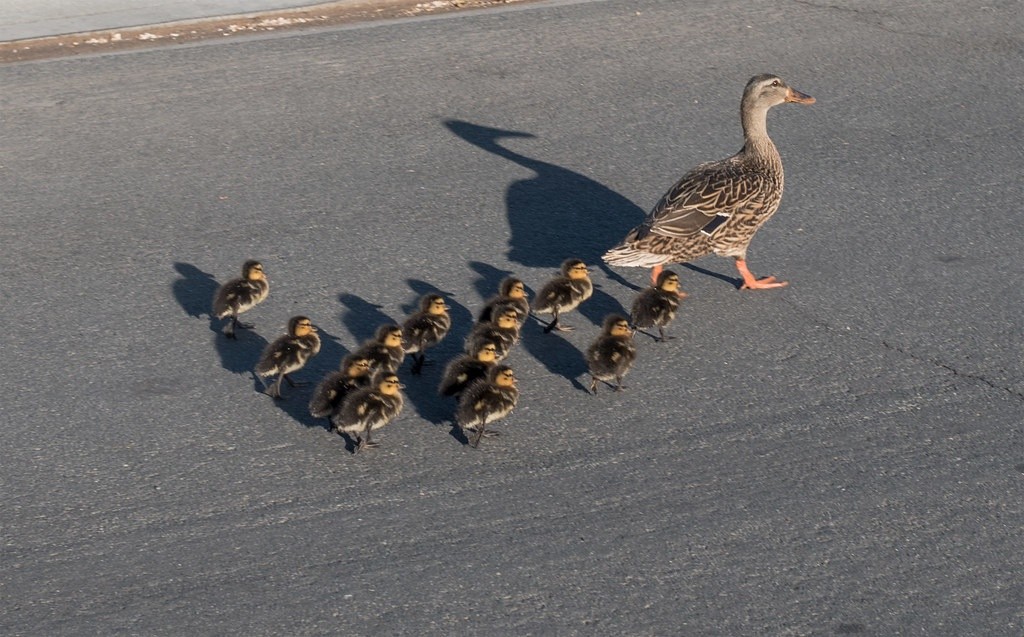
[603,73,817,289]
[212,260,682,456]
